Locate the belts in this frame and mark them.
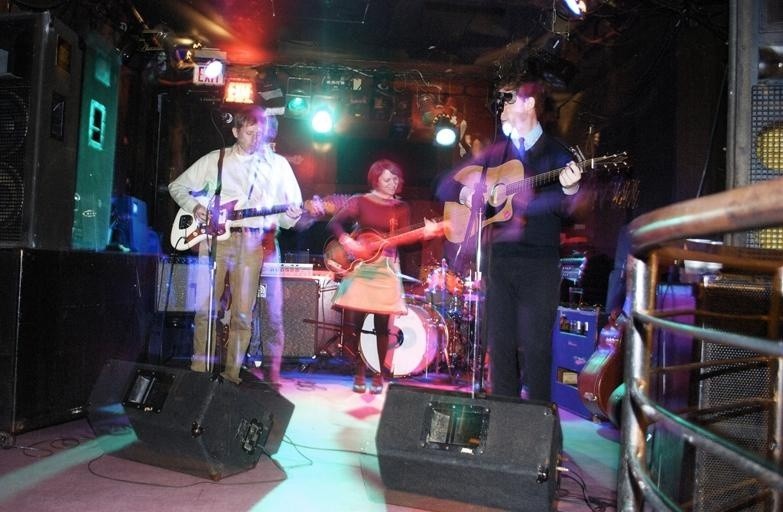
[230,227,260,233]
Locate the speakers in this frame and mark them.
[0,9,119,251]
[84,361,275,481]
[375,383,564,512]
[553,304,611,423]
[255,275,319,359]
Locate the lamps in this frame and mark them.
[431,116,460,153]
[282,76,346,144]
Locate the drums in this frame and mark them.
[356,294,448,379]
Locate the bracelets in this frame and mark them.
[340,237,351,245]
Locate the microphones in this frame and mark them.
[210,109,233,124]
[496,89,518,104]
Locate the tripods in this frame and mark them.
[430,287,485,383]
[299,284,362,375]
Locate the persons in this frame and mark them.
[576,205,680,353]
[433,80,583,406]
[325,159,438,397]
[165,105,304,385]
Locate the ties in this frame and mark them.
[519,137,525,165]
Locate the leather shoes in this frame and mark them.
[352,373,366,393]
[370,375,384,395]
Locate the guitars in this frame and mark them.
[442,146,629,244]
[576,307,624,420]
[169,195,360,250]
[322,218,444,276]
